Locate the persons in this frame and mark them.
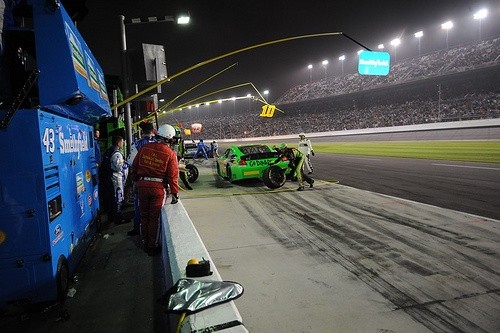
[344,86,436,130]
[426,73,500,122]
[271,144,314,191]
[132,124,179,257]
[271,105,347,136]
[93,123,156,238]
[180,111,270,139]
[210,141,220,159]
[192,139,209,160]
[424,37,500,76]
[297,132,314,174]
[347,54,429,90]
[275,73,354,103]
[178,139,185,158]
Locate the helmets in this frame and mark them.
[280,144,287,150]
[158,125,175,140]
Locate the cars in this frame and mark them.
[184,142,202,157]
[216,144,289,185]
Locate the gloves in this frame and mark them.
[171,194,178,204]
[312,152,314,156]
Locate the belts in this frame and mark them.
[140,176,163,182]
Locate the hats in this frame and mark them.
[299,132,305,137]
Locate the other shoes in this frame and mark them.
[297,186,304,191]
[310,180,314,188]
[128,231,140,236]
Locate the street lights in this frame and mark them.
[390,38,401,64]
[321,59,331,82]
[474,8,489,41]
[441,20,455,49]
[338,54,347,76]
[307,64,313,83]
[413,30,424,56]
